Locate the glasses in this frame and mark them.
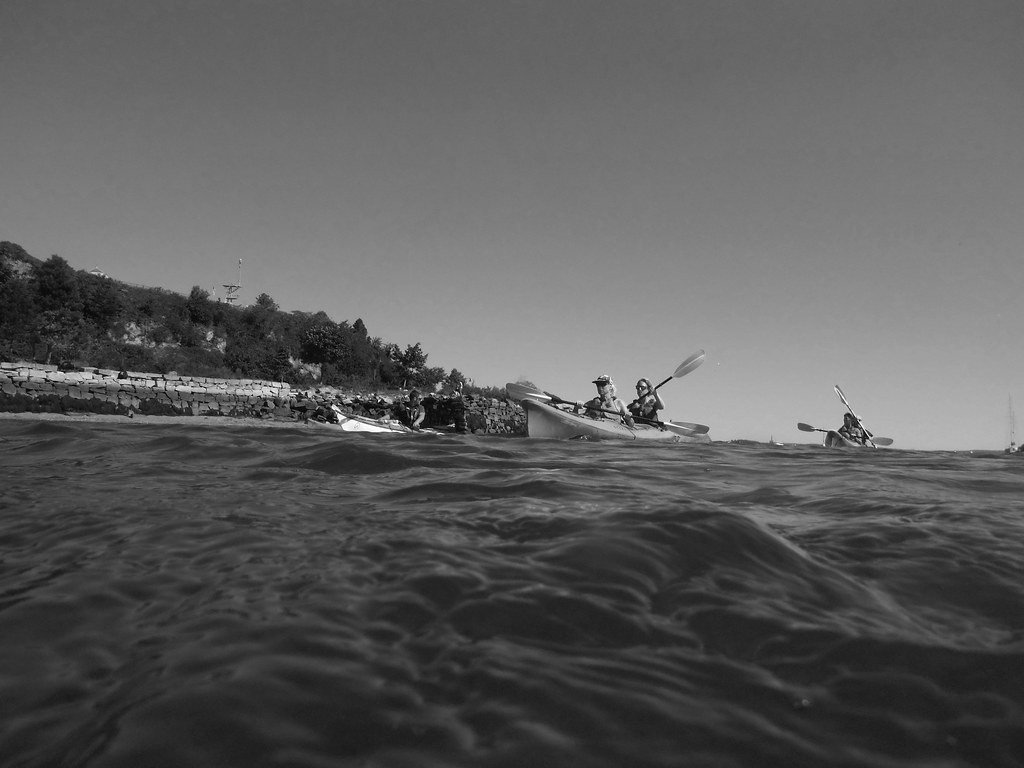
[635,386,648,390]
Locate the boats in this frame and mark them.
[519,397,714,446]
[821,428,866,448]
[330,403,447,436]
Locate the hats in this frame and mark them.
[592,374,613,383]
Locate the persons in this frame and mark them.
[854,415,873,446]
[376,389,425,431]
[837,413,862,445]
[573,374,635,428]
[626,378,665,429]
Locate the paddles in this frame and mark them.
[505,382,711,439]
[796,422,894,446]
[613,348,707,418]
[831,384,881,452]
